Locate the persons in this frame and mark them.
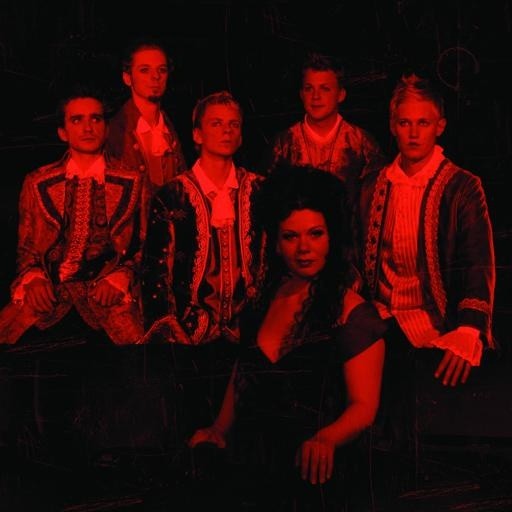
[268,55,384,187]
[186,163,387,506]
[10,41,495,471]
[99,47,187,187]
